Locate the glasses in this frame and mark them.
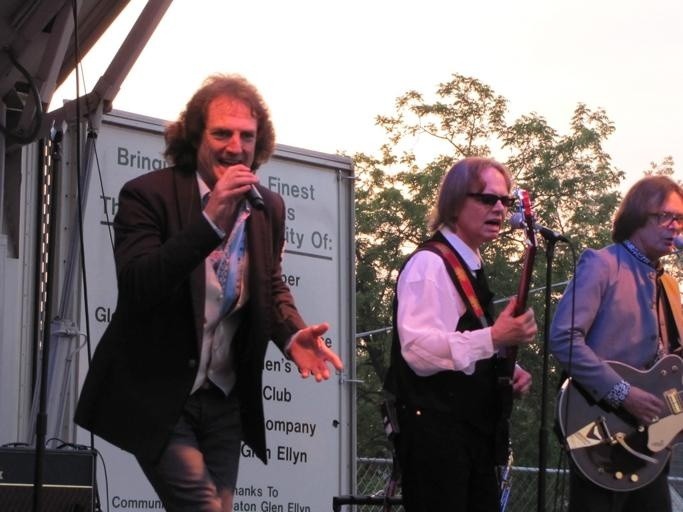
[468,193,516,207]
[648,211,683,229]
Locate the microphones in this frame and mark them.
[243,183,266,211]
[510,212,570,243]
[673,234,682,251]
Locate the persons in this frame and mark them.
[383,158,537,511]
[73,74,344,511]
[550,175,683,511]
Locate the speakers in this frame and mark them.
[0,447,97,511]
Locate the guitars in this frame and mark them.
[479,183,537,467]
[554,350,683,493]
